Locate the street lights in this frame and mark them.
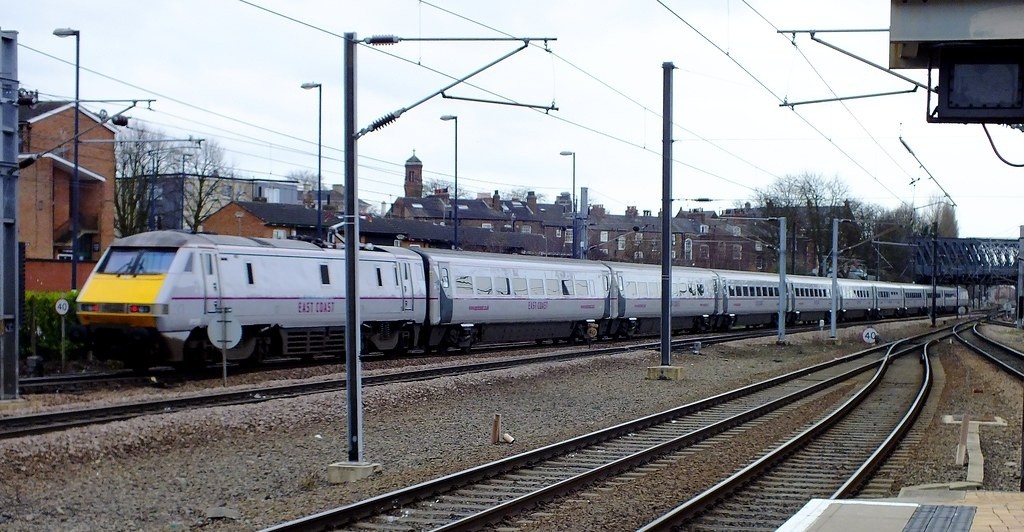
[828,218,852,338]
[300,83,322,225]
[560,151,576,258]
[767,217,788,342]
[51,27,80,293]
[439,114,457,250]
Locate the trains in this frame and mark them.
[75,227,970,378]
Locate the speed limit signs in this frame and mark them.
[54,298,69,316]
